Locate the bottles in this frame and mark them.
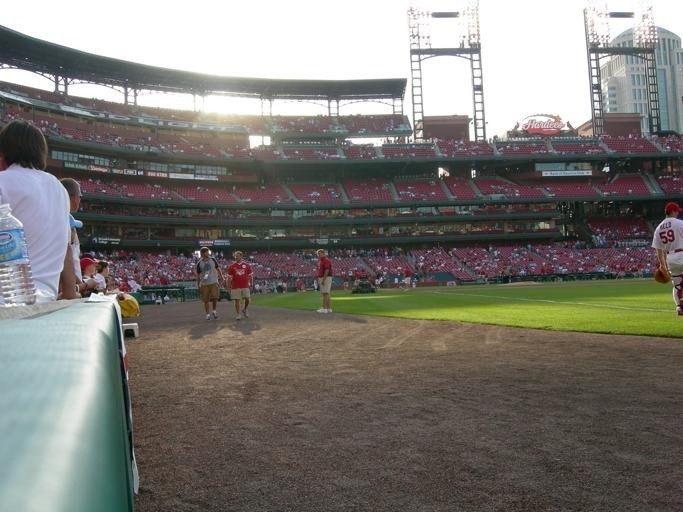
[0,203,36,309]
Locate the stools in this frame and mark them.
[123,323,139,338]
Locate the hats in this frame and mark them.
[79,256,97,268]
[664,202,683,212]
[68,213,84,230]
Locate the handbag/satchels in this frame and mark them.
[118,291,142,319]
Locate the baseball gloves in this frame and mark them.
[654,267,671,283]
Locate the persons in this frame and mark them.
[0,115,683,321]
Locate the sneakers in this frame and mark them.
[313,307,333,314]
[205,310,249,321]
